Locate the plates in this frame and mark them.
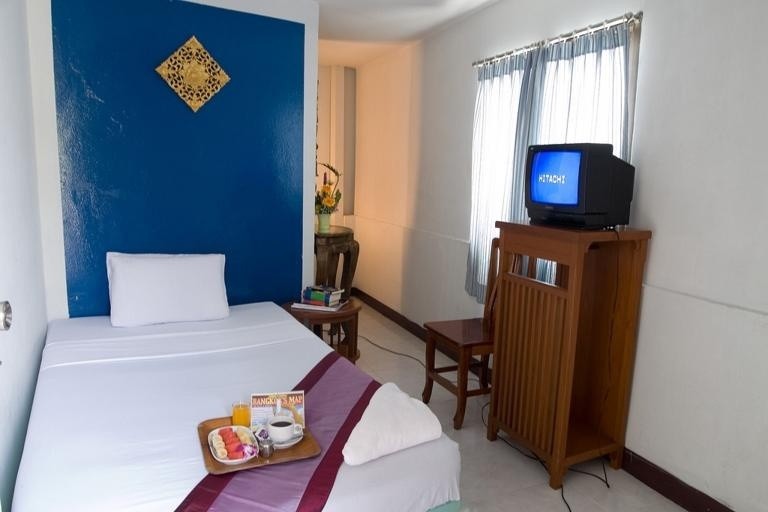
[208,425,259,465]
[255,423,304,449]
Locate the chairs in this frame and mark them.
[420,237,537,430]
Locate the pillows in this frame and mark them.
[105,249,231,328]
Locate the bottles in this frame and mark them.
[258,440,275,458]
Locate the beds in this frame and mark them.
[8,300,461,511]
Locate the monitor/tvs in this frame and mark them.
[525,143,636,232]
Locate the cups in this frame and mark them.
[267,416,303,443]
[232,400,252,427]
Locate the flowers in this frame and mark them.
[315,160,344,215]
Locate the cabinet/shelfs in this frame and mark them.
[486,219,652,490]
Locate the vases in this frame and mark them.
[317,214,330,232]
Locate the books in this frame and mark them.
[291,284,349,312]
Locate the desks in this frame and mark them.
[314,224,359,335]
[282,298,362,366]
